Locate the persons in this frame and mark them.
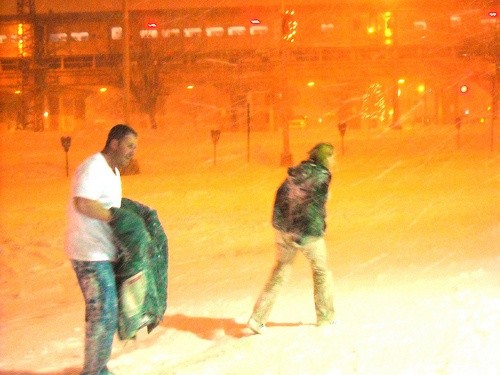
[65,124,153,375]
[247,142,336,334]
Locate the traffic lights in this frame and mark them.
[457,79,471,94]
